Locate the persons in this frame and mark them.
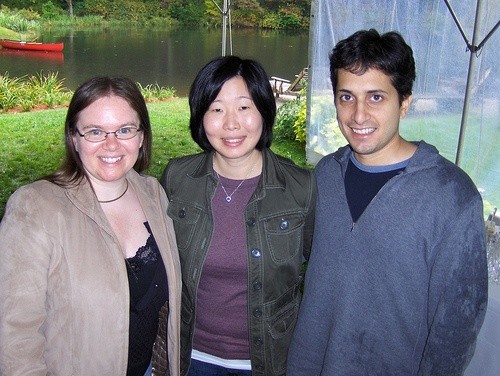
[287,30,488,376]
[1,75,183,376]
[157,55,317,376]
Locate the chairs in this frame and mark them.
[270,68,309,99]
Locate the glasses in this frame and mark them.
[76,126,142,143]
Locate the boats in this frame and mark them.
[0,38,64,51]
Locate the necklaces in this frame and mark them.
[98,179,130,204]
[213,160,260,203]
